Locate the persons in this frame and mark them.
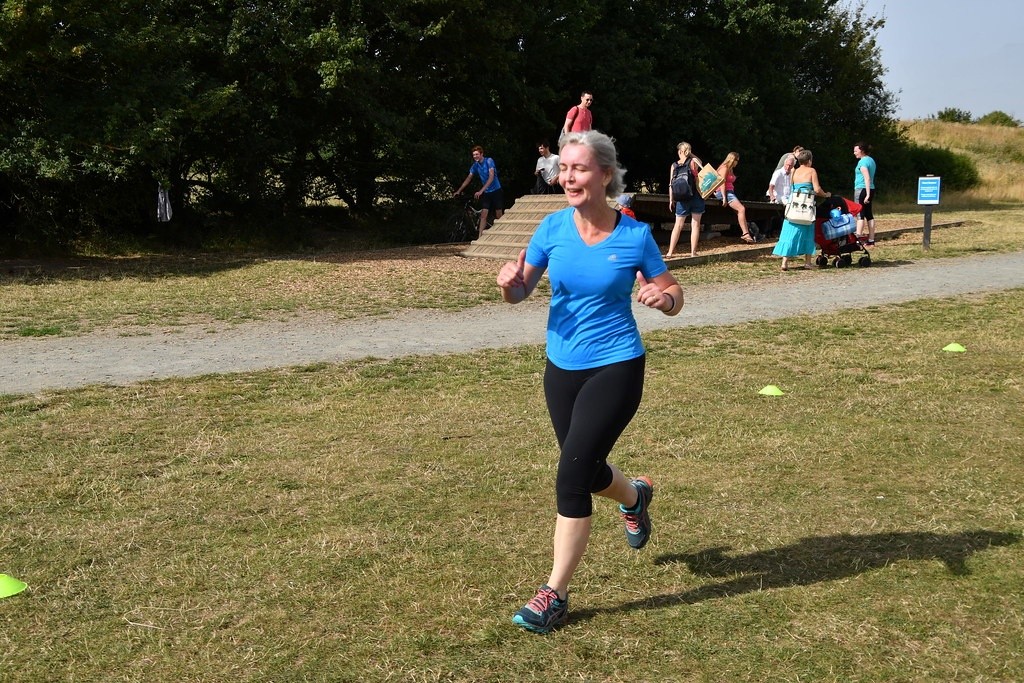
[616,195,635,219]
[497,131,684,634]
[454,146,503,238]
[662,142,705,259]
[853,143,876,245]
[563,91,593,136]
[534,141,560,194]
[766,146,831,271]
[712,152,753,242]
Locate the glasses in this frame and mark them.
[583,98,593,102]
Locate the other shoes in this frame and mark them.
[741,232,756,243]
[864,240,874,245]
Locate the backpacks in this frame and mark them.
[670,158,696,201]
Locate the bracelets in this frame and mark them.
[662,293,675,313]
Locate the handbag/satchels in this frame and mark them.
[822,212,857,240]
[557,129,565,146]
[692,157,726,200]
[784,186,815,226]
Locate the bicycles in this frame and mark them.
[444,192,496,243]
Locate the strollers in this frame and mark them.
[814,194,871,269]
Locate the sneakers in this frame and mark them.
[619,476,654,549]
[512,584,568,633]
